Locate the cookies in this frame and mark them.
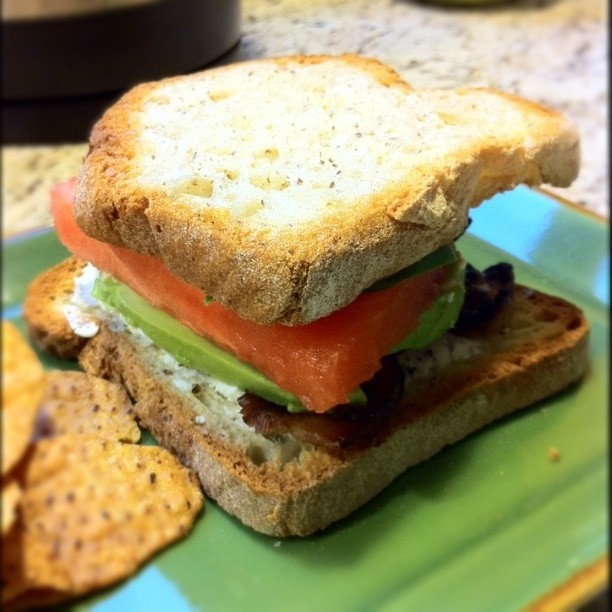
[0,317,203,612]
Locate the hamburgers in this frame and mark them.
[25,54,589,539]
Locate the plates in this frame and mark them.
[1,179,608,611]
